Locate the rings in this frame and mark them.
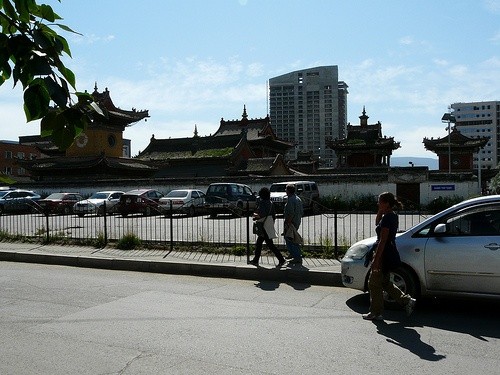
[373,270,376,271]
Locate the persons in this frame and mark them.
[284,184,302,264]
[248,187,286,267]
[362,192,416,320]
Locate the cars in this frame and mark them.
[35,192,85,215]
[119,188,167,217]
[340,196,500,313]
[72,190,126,217]
[157,188,209,217]
[0,186,42,215]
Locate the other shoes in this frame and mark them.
[276,259,287,267]
[362,311,383,322]
[248,258,259,266]
[405,296,416,318]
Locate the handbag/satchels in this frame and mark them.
[380,243,402,272]
[256,221,264,230]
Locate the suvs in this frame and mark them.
[202,182,263,218]
[268,180,321,217]
